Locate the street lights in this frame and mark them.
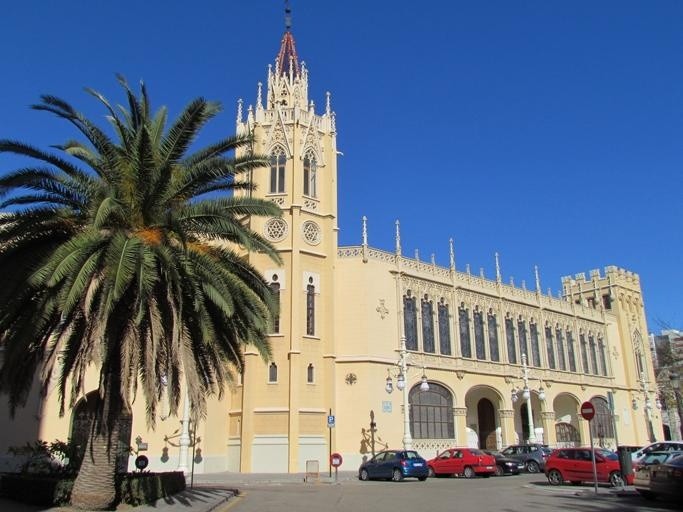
[511,353,544,446]
[632,372,663,445]
[385,334,430,449]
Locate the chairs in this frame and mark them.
[305,460,320,483]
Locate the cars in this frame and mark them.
[425,448,498,479]
[545,446,637,487]
[503,444,552,473]
[616,441,682,509]
[358,450,428,482]
[481,450,524,477]
